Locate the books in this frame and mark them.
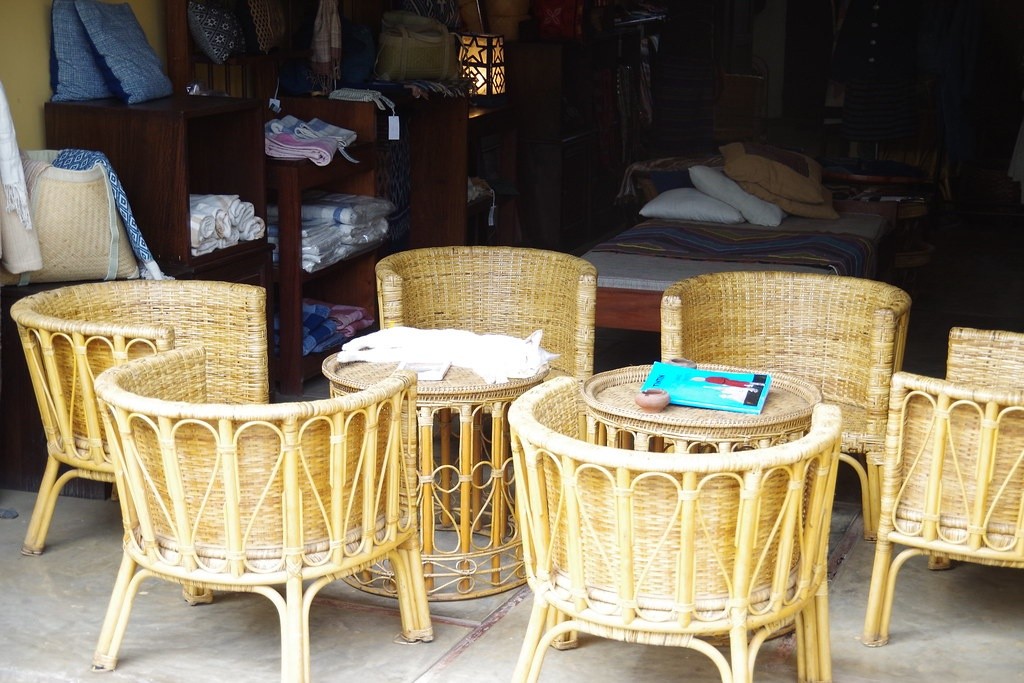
[641,362,772,415]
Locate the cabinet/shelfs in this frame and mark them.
[268,93,471,395]
[506,35,646,250]
[270,140,380,395]
[43,92,275,404]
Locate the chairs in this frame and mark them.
[90,342,436,683]
[10,278,275,554]
[494,371,844,683]
[656,269,915,541]
[863,325,1024,650]
[373,245,596,380]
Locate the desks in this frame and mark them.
[584,358,825,644]
[321,349,549,603]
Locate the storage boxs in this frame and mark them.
[713,68,770,145]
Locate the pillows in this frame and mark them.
[717,140,825,206]
[397,0,467,33]
[638,187,746,224]
[237,0,289,55]
[185,0,246,66]
[535,0,576,41]
[49,0,114,102]
[688,165,789,227]
[738,180,842,222]
[75,0,174,105]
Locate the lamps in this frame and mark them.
[458,31,512,109]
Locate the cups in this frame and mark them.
[636,389,670,413]
[665,358,697,369]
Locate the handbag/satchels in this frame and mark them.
[373,9,469,82]
[0,148,141,286]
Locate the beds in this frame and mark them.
[582,199,898,334]
